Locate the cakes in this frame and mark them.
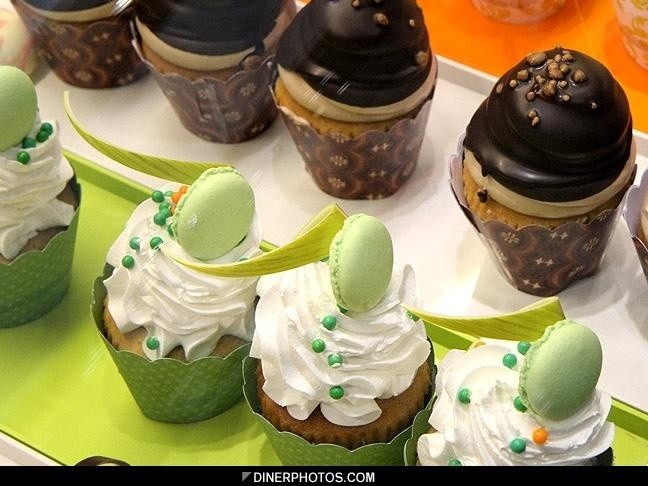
[168,203,438,466]
[268,0,438,199]
[0,66,82,329]
[403,293,617,466]
[64,91,266,422]
[10,0,146,88]
[130,0,297,143]
[449,47,637,297]
